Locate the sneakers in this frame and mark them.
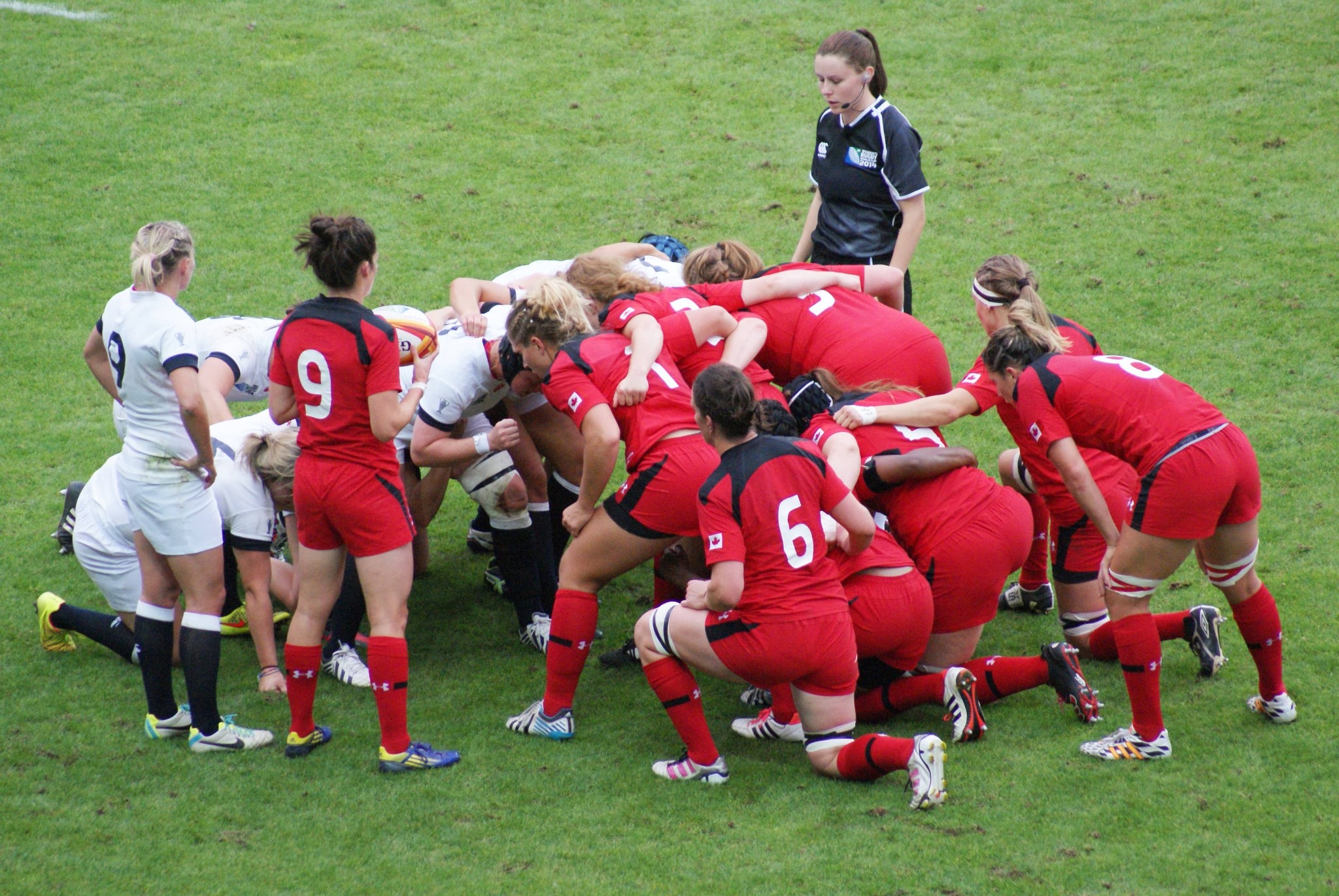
[1189,605,1230,682]
[220,604,291,636]
[484,557,511,598]
[506,699,575,739]
[1247,692,1297,723]
[652,755,729,784]
[464,519,495,554]
[145,705,191,741]
[598,640,639,667]
[51,481,86,554]
[285,725,331,758]
[378,741,462,773]
[187,715,274,754]
[997,583,1055,612]
[943,666,988,743]
[518,611,552,654]
[732,709,804,742]
[740,688,773,707]
[320,643,371,687]
[34,591,77,652]
[908,734,948,811]
[1040,642,1104,723]
[1080,723,1173,759]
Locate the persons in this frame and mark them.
[36,408,299,694]
[84,220,273,752]
[267,209,460,774]
[734,402,987,742]
[505,275,775,740]
[624,255,686,287]
[984,300,1296,759]
[565,252,862,667]
[783,368,1103,725]
[834,256,1229,679]
[793,28,930,315]
[683,238,952,398]
[52,314,289,561]
[320,301,553,686]
[448,241,670,604]
[635,362,948,809]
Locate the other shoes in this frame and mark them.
[594,631,605,642]
[273,512,289,560]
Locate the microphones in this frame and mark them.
[839,77,866,112]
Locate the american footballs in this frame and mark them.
[371,305,438,367]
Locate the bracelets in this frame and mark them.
[473,432,490,455]
[407,382,427,398]
